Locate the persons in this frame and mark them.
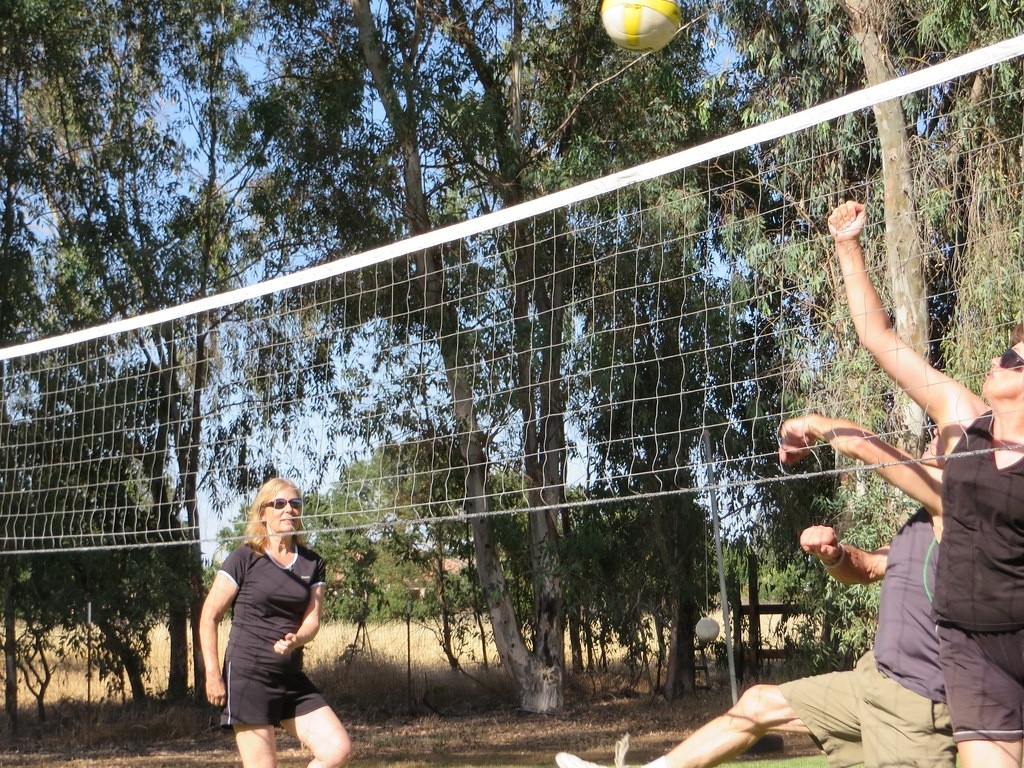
[556,413,956,768]
[827,201,1024,767]
[198,478,352,767]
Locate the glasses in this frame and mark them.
[261,498,301,509]
[1000,348,1024,372]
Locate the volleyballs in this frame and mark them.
[600,0,682,54]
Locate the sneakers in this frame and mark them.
[556,733,629,768]
